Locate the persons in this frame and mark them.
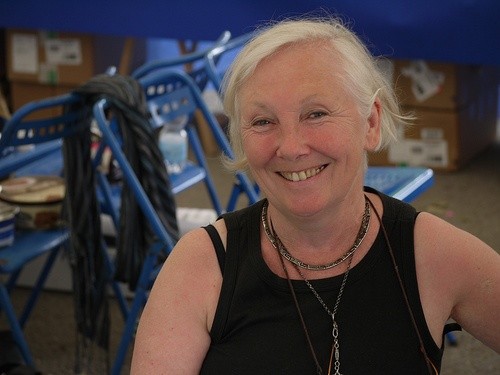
[127,9,500,375]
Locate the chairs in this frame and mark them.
[0,28,460,374]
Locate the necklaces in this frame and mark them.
[258,192,372,271]
[267,192,370,375]
